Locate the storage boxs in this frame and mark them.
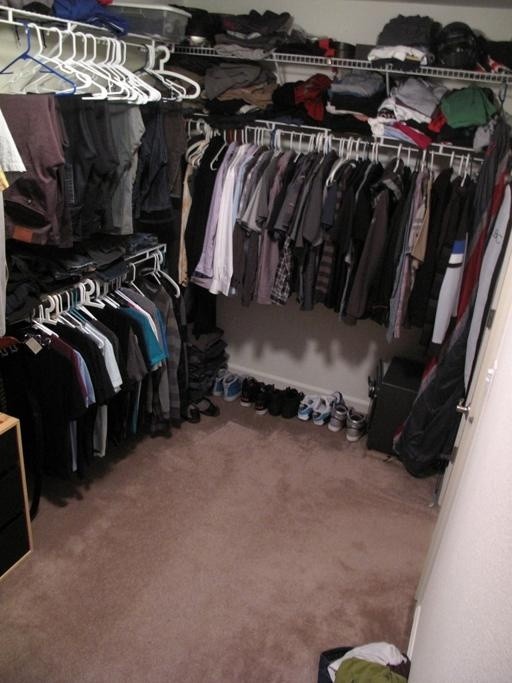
[107,3,191,43]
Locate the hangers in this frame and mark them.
[1,21,202,103]
[0,250,182,349]
[184,117,480,176]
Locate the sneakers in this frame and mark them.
[269,386,305,418]
[312,391,342,426]
[297,394,319,421]
[180,387,219,423]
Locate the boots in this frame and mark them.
[346,409,370,442]
[211,377,223,397]
[254,385,275,416]
[239,377,264,407]
[328,403,348,433]
[223,374,243,403]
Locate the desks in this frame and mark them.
[365,353,463,464]
